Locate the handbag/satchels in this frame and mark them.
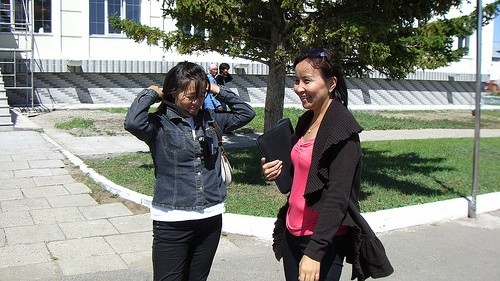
[221,151,234,186]
[256,117,295,194]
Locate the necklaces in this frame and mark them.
[309,122,321,133]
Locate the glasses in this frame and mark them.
[300,47,335,71]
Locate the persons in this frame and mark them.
[124,63,256,281]
[261,47,395,281]
[204,63,233,135]
